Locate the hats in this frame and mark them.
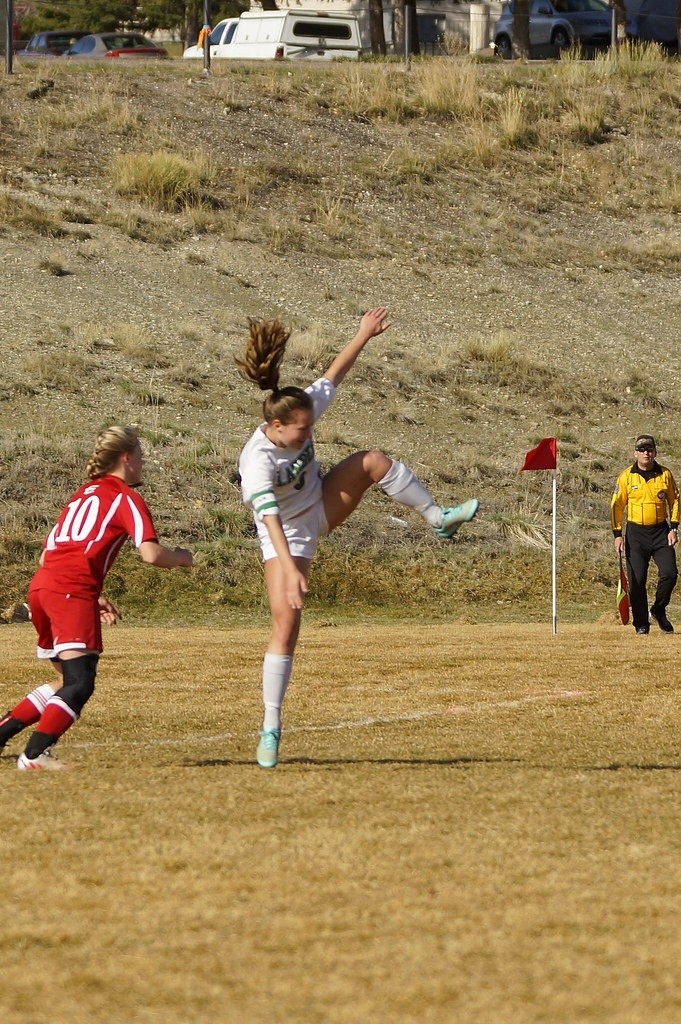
[635,435,655,448]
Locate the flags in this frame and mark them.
[617,568,630,625]
[521,438,557,470]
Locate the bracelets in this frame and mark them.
[672,529,678,533]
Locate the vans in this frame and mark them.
[183,10,362,60]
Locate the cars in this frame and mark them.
[493,0,681,60]
[16,31,170,58]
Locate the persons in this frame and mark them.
[233,307,480,768]
[611,435,681,635]
[0,425,193,770]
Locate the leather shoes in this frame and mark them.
[650,605,674,633]
[636,626,649,634]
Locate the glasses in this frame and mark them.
[635,447,656,452]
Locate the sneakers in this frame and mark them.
[433,498,478,538]
[256,728,281,767]
[16,743,65,772]
